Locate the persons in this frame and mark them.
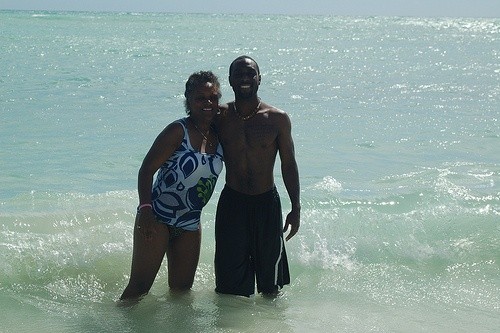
[211,55,301,299]
[119,69,223,305]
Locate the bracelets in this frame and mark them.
[138,203,153,210]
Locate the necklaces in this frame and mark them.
[192,119,214,146]
[232,97,262,119]
